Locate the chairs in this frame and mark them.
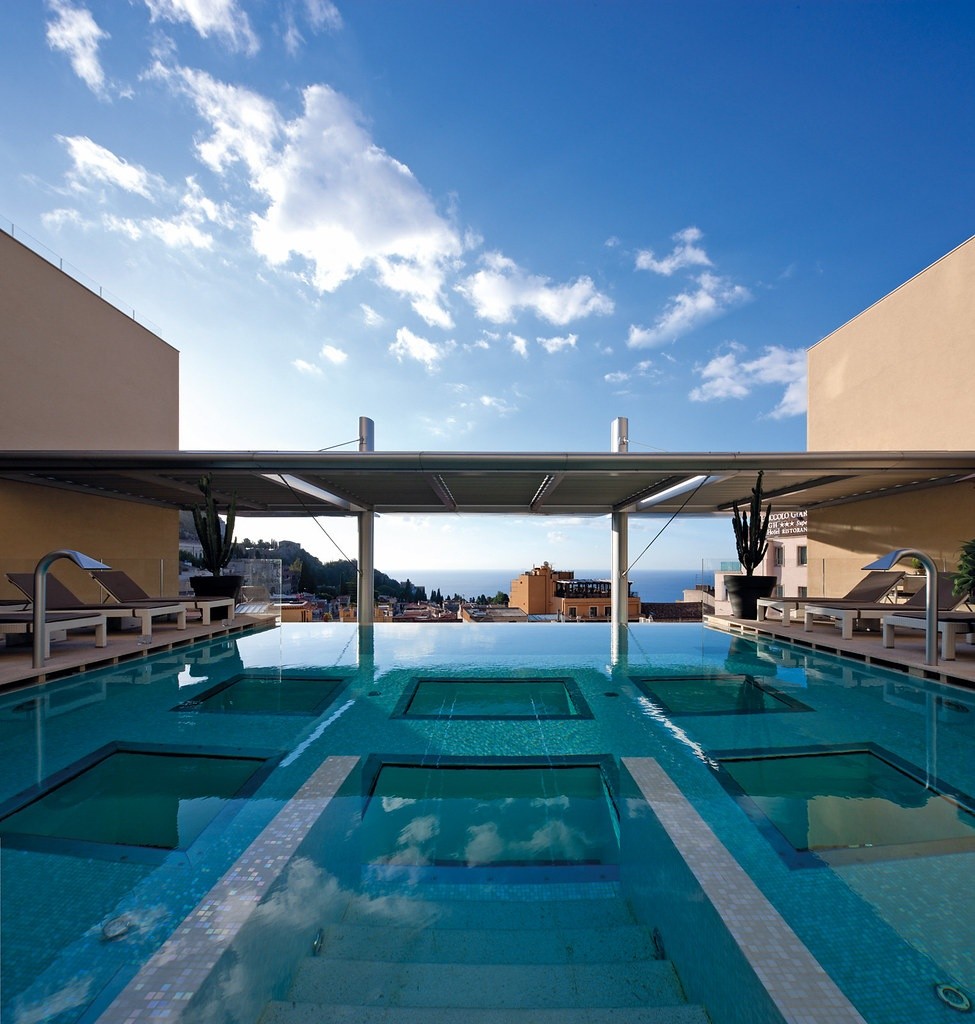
[3,570,186,637]
[0,612,107,658]
[87,569,235,626]
[755,570,904,626]
[879,612,974,661]
[805,571,972,638]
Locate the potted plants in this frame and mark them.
[723,471,778,620]
[178,470,245,621]
